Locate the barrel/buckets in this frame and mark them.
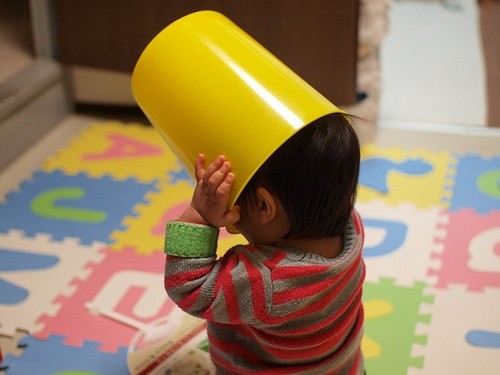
[131,10,351,232]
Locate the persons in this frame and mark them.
[164,112,366,375]
